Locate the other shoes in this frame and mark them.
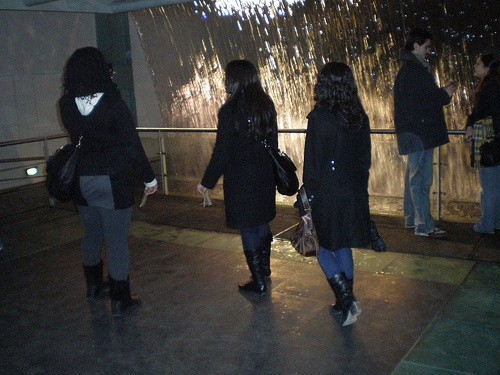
[474,225,495,233]
[406,224,448,238]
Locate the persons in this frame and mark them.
[464,52,500,234]
[58,47,158,317]
[197,60,278,298]
[293,61,372,327]
[394,28,456,238]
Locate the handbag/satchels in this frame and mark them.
[269,146,299,196]
[45,142,80,204]
[289,185,320,256]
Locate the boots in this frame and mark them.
[82,258,109,303]
[238,233,272,293]
[329,271,362,326]
[106,273,142,321]
